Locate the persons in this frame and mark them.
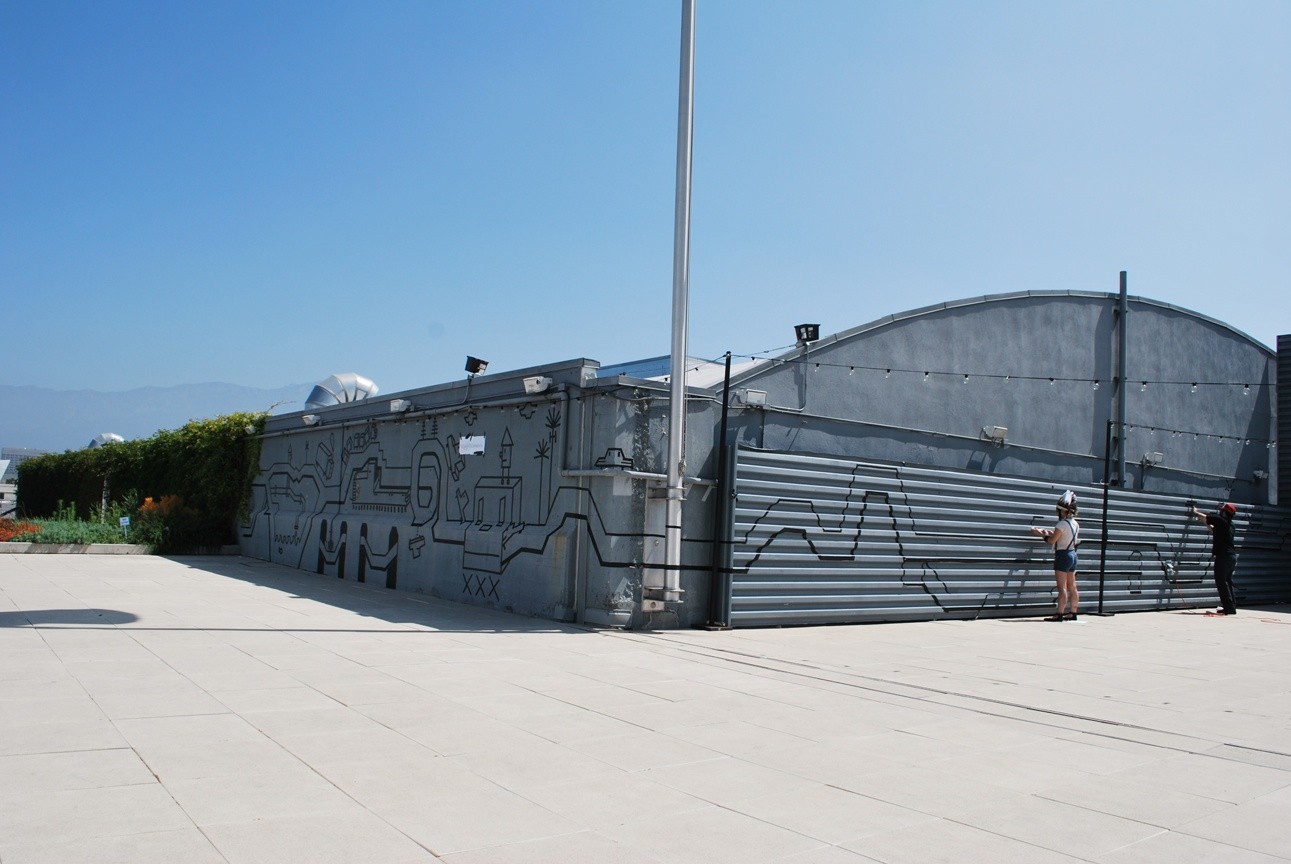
[1192,502,1237,614]
[1032,490,1080,622]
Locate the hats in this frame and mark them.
[1220,504,1237,513]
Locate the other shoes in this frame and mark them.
[1218,608,1237,614]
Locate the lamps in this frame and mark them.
[1254,470,1266,479]
[522,376,554,395]
[244,426,258,435]
[466,356,489,376]
[983,426,1008,448]
[1153,452,1164,463]
[302,414,321,426]
[389,399,413,414]
[794,322,821,341]
[746,389,767,405]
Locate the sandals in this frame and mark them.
[1044,613,1064,621]
[1064,613,1078,621]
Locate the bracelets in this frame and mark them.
[1044,535,1050,542]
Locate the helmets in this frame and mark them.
[1056,490,1078,514]
